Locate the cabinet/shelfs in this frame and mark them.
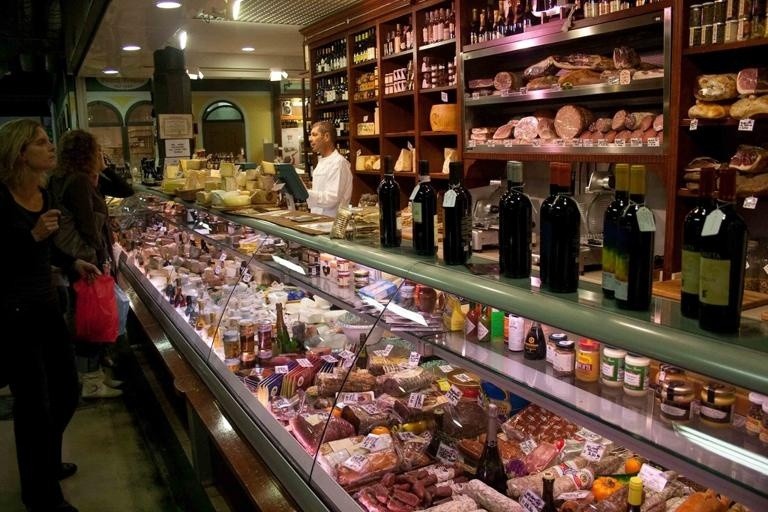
[106,181,768,512]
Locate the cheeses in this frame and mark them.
[164,159,279,208]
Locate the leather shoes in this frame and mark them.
[55,462,78,481]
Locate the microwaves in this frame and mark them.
[274,302,294,353]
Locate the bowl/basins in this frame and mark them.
[162,159,278,208]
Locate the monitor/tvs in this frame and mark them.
[273,161,310,203]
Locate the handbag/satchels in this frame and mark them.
[73,270,131,343]
[48,171,108,266]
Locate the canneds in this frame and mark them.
[545,332,768,451]
[222,319,273,375]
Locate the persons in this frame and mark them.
[94,144,135,368]
[0,117,102,512]
[305,122,353,218]
[56,128,125,404]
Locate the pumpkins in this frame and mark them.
[592,476,623,502]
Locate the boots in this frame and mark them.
[77,368,125,398]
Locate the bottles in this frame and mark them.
[600,163,655,313]
[312,78,353,167]
[357,332,369,370]
[476,402,508,492]
[627,477,642,511]
[537,161,581,295]
[498,160,531,283]
[310,7,457,76]
[678,168,749,336]
[599,345,632,391]
[222,221,258,252]
[447,366,484,437]
[139,156,162,185]
[525,321,546,359]
[746,392,768,446]
[379,159,403,252]
[471,1,661,45]
[441,161,474,267]
[412,159,439,257]
[165,276,222,347]
[624,356,649,396]
[539,472,558,511]
